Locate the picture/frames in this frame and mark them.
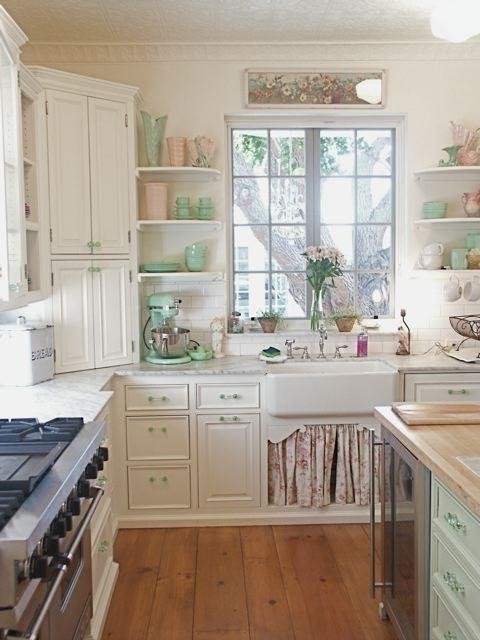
[244,68,386,108]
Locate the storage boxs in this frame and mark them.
[1,320,54,387]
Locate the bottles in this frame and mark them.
[229,312,243,333]
[242,312,251,334]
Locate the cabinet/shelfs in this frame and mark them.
[194,382,263,513]
[135,165,225,346]
[90,444,113,613]
[43,83,134,368]
[14,87,44,292]
[424,474,478,639]
[405,374,480,411]
[117,379,193,512]
[412,158,478,273]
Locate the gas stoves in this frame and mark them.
[0,415,116,609]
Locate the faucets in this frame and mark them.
[318,329,329,357]
[284,339,296,357]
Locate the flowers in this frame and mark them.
[306,248,342,293]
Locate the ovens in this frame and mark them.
[0,482,107,638]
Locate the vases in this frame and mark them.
[309,290,324,328]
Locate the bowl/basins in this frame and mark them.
[189,352,212,361]
[185,243,208,272]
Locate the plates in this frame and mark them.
[175,216,194,219]
[421,202,446,219]
[196,216,213,220]
[175,206,193,209]
[142,263,179,271]
[196,206,214,208]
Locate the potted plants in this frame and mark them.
[335,311,357,332]
[258,314,279,333]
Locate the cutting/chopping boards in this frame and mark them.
[391,403,480,425]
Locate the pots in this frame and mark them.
[151,326,190,358]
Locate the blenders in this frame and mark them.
[141,294,194,364]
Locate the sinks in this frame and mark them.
[268,361,390,375]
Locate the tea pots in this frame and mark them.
[461,192,480,217]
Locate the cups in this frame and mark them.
[199,197,212,206]
[199,208,213,216]
[144,183,168,220]
[463,274,480,302]
[418,255,443,268]
[442,274,462,302]
[175,197,190,206]
[171,208,189,215]
[451,248,469,270]
[423,243,443,254]
[166,137,186,167]
